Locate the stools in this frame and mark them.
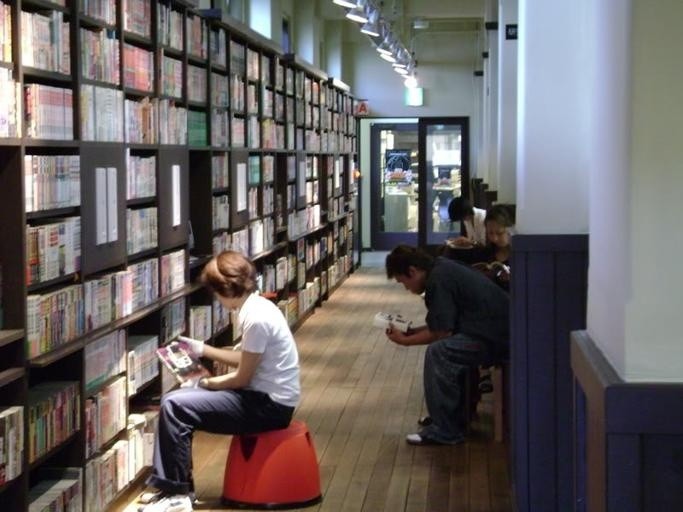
[457,362,507,443]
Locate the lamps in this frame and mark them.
[330,0,418,89]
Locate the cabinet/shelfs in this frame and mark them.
[0,1,361,511]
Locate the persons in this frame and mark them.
[385,244,509,445]
[469,204,514,293]
[443,195,485,257]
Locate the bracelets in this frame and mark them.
[473,240,479,247]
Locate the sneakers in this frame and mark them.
[140,489,197,504]
[137,496,193,512]
[404,433,442,445]
[478,374,492,394]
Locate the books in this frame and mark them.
[472,261,508,280]
[443,239,472,250]
[373,311,412,333]
[0,0,367,512]
